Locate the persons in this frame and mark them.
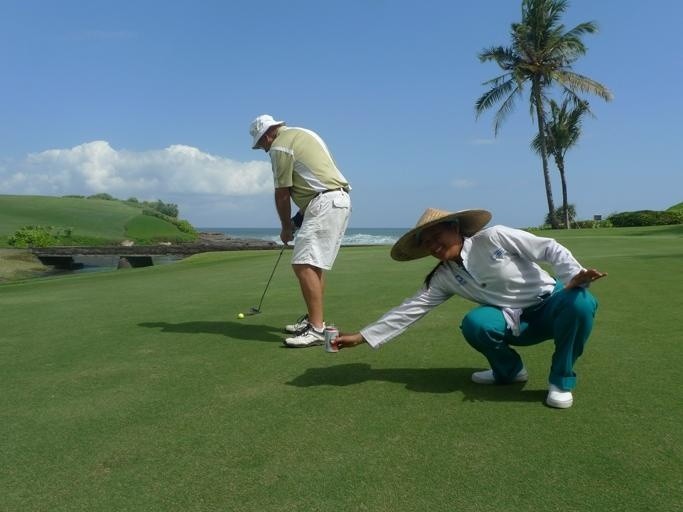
[330,206,608,409]
[248,113,352,348]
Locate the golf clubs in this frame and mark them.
[250,241,287,312]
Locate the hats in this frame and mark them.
[390,207,493,262]
[250,115,285,150]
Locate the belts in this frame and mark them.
[314,188,349,197]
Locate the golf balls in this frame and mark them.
[238,313,244,319]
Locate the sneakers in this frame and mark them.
[472,364,529,385]
[546,384,573,408]
[285,315,326,347]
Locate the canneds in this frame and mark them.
[325,326,339,353]
